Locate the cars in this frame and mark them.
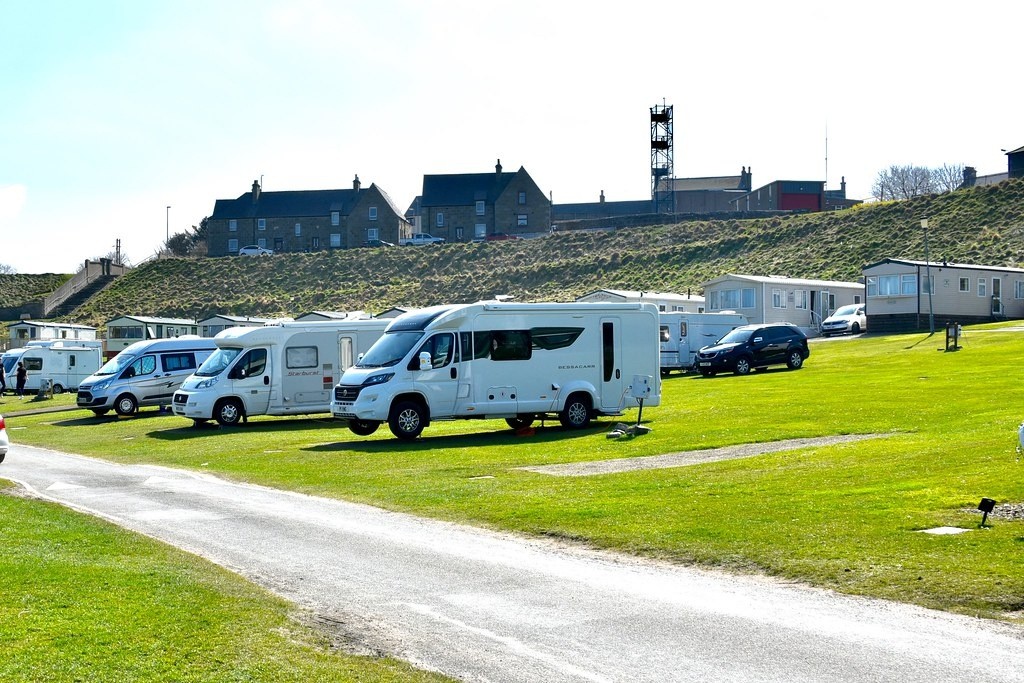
[238,245,273,257]
[484,231,519,241]
[361,238,395,248]
[821,302,866,338]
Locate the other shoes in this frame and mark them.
[1,393,3,398]
[19,395,24,400]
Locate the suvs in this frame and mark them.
[693,322,810,378]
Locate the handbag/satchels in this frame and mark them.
[24,370,29,381]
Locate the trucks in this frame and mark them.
[170,317,399,428]
[0,339,104,394]
[659,310,748,374]
[330,296,662,440]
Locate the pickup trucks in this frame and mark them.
[398,231,446,248]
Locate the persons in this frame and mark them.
[17,362,28,400]
[0,357,7,397]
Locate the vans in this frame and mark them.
[76,334,237,416]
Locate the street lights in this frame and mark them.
[166,205,172,258]
[919,216,935,333]
